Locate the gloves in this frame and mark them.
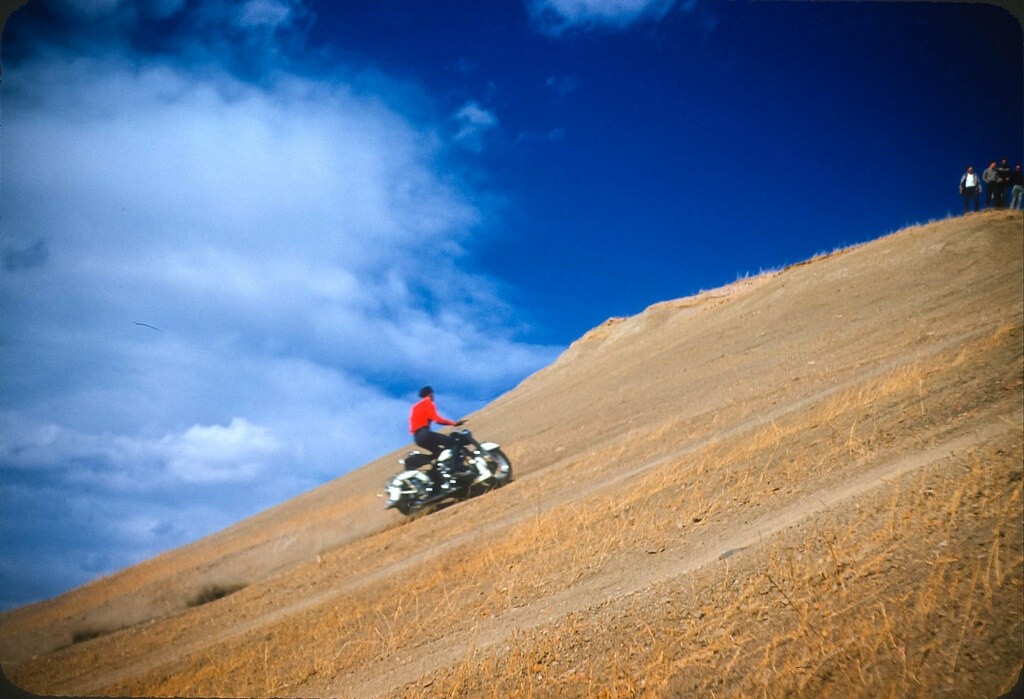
[455,419,467,427]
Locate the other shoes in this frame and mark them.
[455,467,471,476]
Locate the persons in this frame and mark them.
[410,385,468,472]
[960,159,1024,212]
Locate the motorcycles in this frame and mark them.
[384,419,513,518]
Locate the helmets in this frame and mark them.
[418,387,432,397]
